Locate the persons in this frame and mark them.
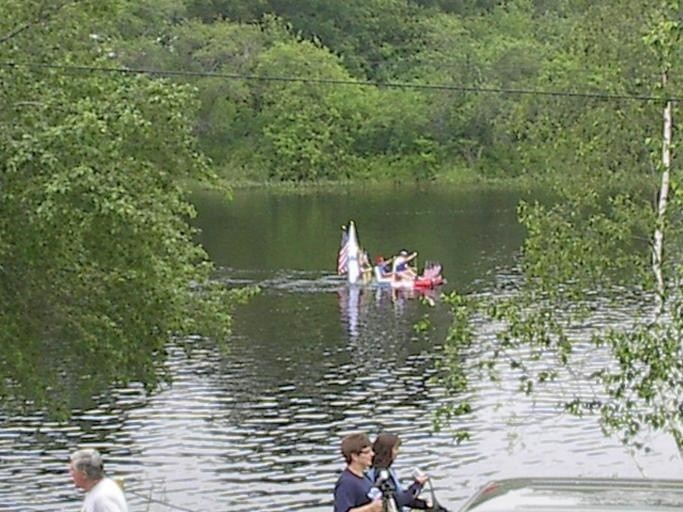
[394,248,421,279]
[69,448,129,512]
[330,433,385,512]
[376,255,393,276]
[367,431,428,512]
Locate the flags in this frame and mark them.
[337,231,369,275]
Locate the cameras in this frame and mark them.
[411,468,423,478]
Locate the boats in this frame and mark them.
[317,268,448,294]
